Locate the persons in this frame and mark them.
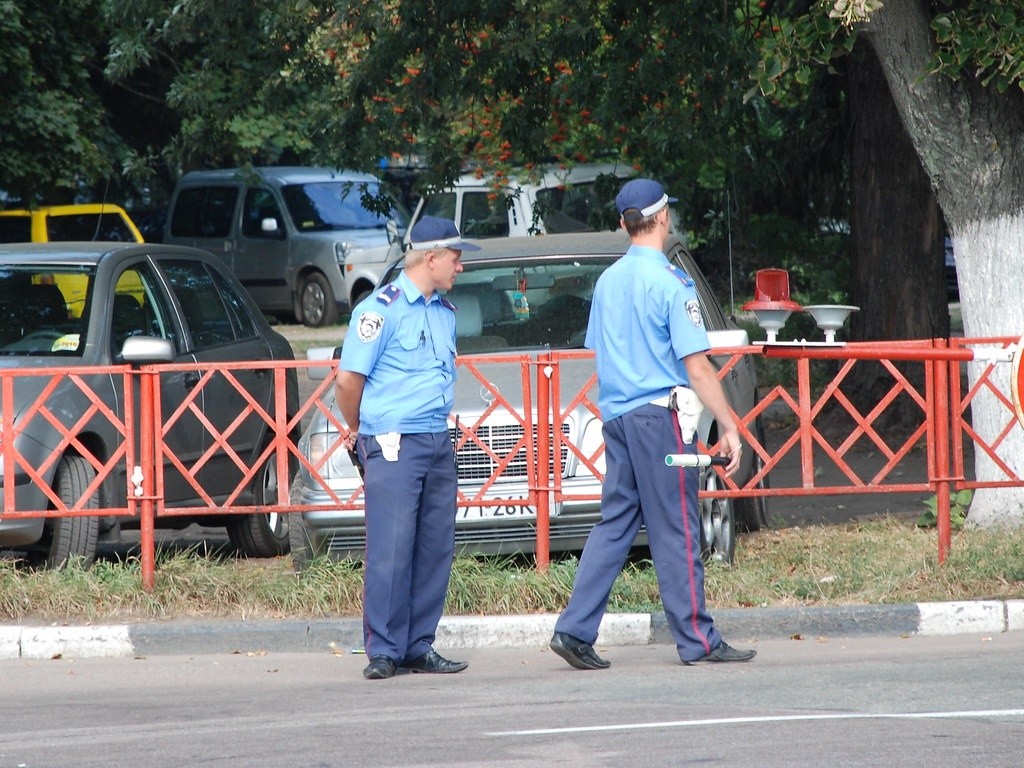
[333,214,471,680]
[549,178,757,669]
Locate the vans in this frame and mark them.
[163,165,411,326]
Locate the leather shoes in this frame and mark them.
[549,633,610,670]
[362,655,395,679]
[687,643,756,663]
[399,649,469,673]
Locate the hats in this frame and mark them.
[615,179,680,219]
[408,215,481,250]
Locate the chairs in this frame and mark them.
[15,283,147,359]
[450,281,587,349]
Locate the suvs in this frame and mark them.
[0,205,154,324]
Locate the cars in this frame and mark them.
[0,241,301,566]
[387,163,643,301]
[288,230,774,567]
